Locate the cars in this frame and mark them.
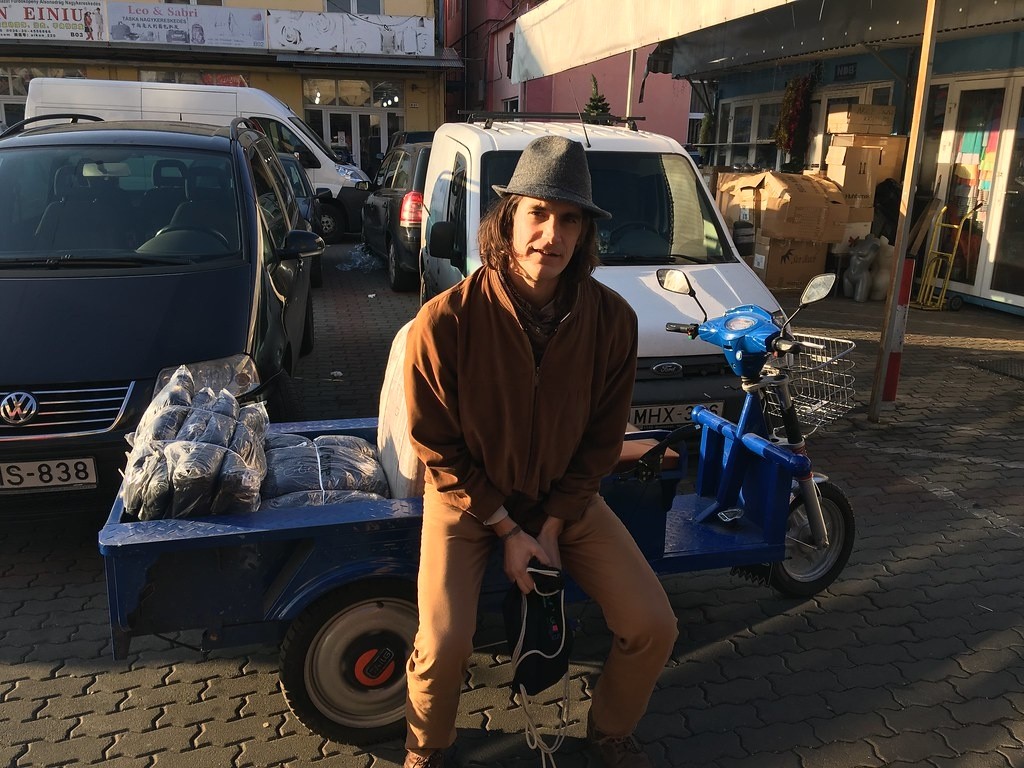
[277,152,332,287]
[0,112,325,511]
[354,142,432,293]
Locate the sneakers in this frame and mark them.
[587,706,651,768]
[403,748,444,768]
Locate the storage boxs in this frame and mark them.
[699,103,909,294]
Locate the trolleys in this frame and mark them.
[909,202,983,311]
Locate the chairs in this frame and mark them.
[35,166,119,249]
[614,422,681,472]
[170,168,238,247]
[145,159,188,202]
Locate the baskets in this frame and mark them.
[762,333,856,428]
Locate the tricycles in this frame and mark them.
[94,266,858,748]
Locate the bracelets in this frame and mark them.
[500,524,521,543]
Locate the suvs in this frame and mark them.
[415,107,796,431]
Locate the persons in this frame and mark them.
[398,135,678,768]
[83,7,104,41]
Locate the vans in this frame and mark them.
[376,129,436,162]
[24,76,373,246]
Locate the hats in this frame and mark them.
[491,136,612,220]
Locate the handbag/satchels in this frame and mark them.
[506,557,570,768]
